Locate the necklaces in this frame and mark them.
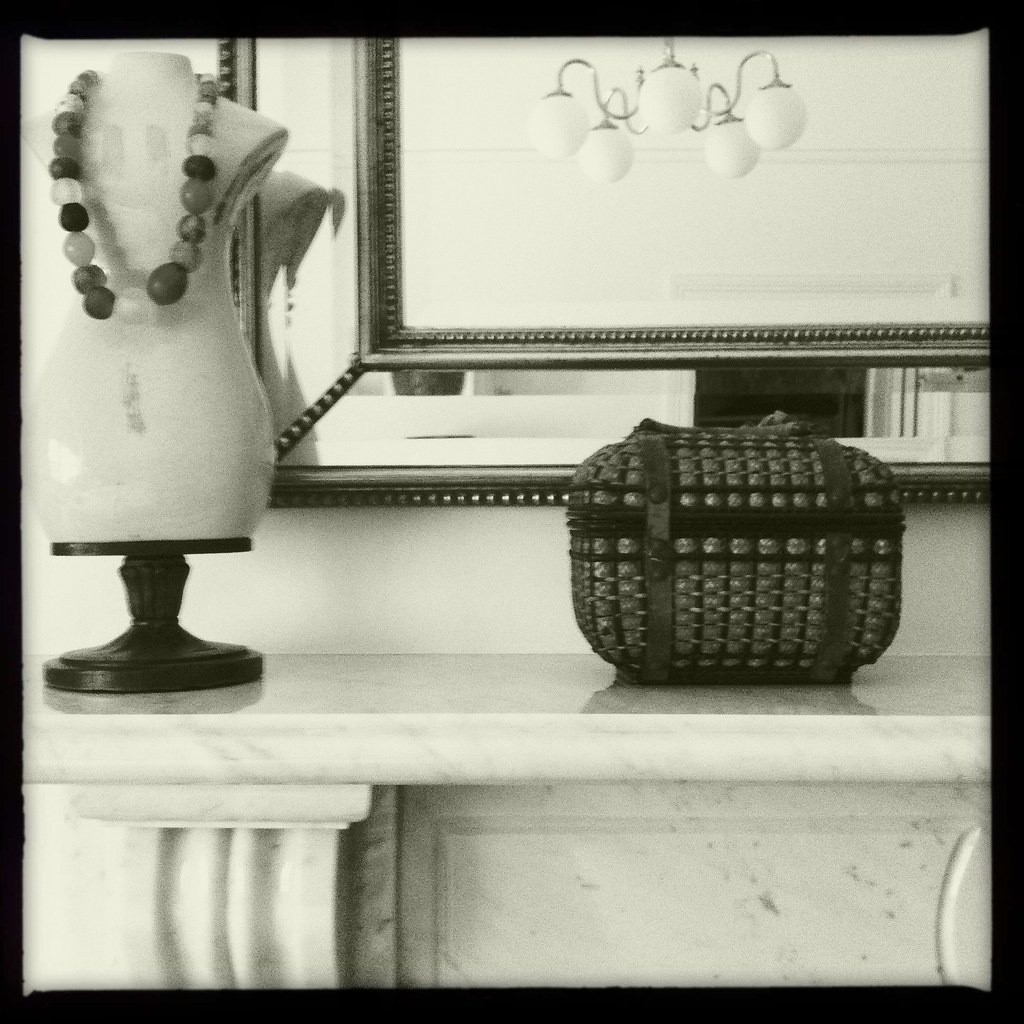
[50,63,217,320]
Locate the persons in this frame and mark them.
[28,53,293,557]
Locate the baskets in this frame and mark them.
[564,415,906,684]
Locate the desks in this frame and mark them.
[22,649,991,991]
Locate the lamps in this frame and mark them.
[532,36,808,185]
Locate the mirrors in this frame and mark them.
[212,39,992,511]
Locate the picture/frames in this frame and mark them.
[352,30,989,374]
